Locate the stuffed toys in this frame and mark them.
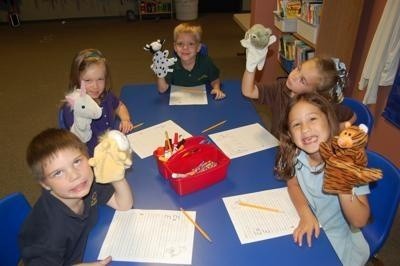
[62,78,104,145]
[87,128,134,187]
[141,37,178,79]
[237,22,278,72]
[318,120,384,195]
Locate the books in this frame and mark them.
[274,0,324,67]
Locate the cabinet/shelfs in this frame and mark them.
[136,0,172,24]
[232,0,393,119]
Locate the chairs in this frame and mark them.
[361,150,400,256]
[0,192,34,266]
[342,97,373,134]
[172,44,208,56]
[59,107,67,129]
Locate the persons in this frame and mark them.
[240,55,358,141]
[17,128,135,266]
[56,46,135,161]
[271,92,372,265]
[156,21,227,102]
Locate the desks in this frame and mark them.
[83,80,344,266]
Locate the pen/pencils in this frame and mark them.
[132,123,144,129]
[171,160,218,179]
[180,207,214,244]
[158,131,192,163]
[200,120,227,135]
[239,202,282,213]
[184,89,207,95]
